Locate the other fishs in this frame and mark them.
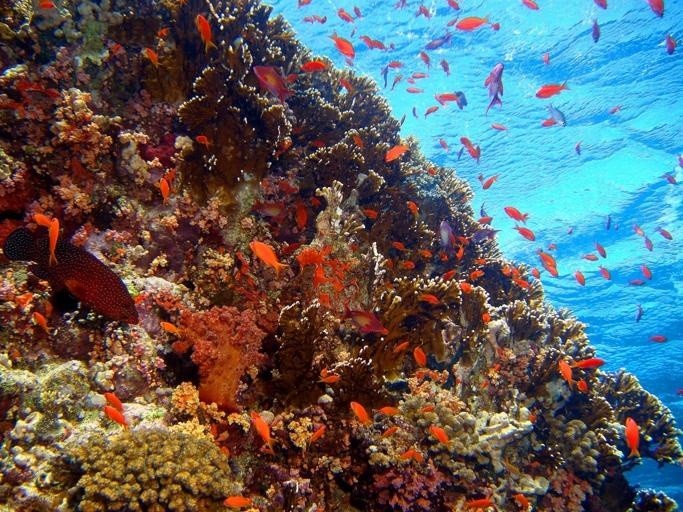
[1,0,683,512]
